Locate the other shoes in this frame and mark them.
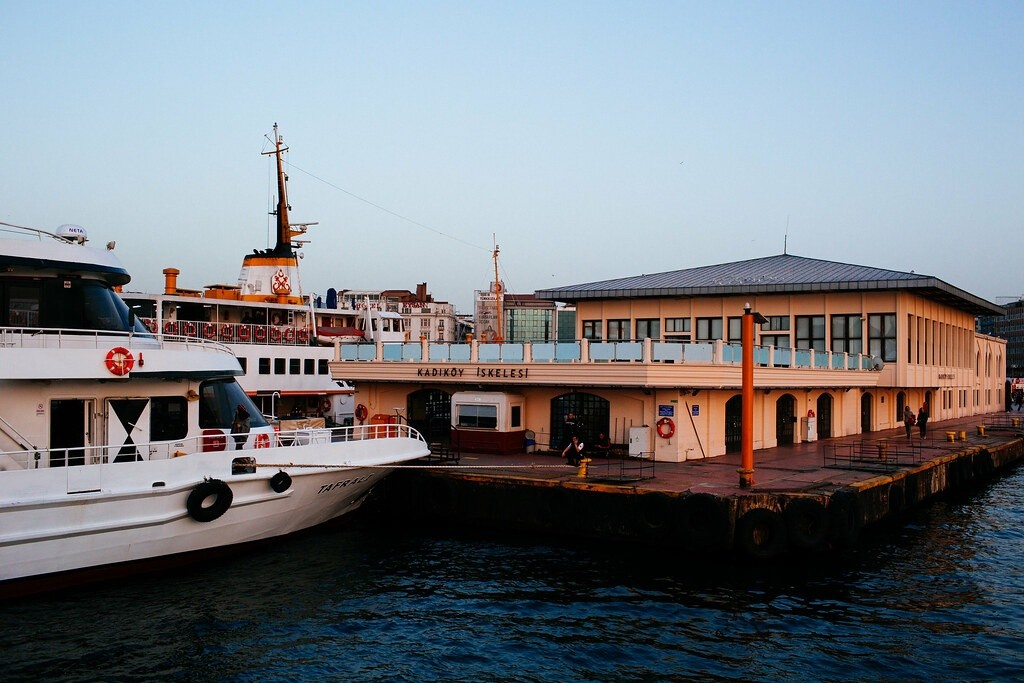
[907,435,910,439]
[923,436,926,439]
[920,437,923,439]
[1007,411,1010,413]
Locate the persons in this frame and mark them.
[230,404,251,450]
[564,412,577,432]
[901,405,917,442]
[917,403,930,440]
[562,436,585,467]
[591,432,612,457]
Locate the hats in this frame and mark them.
[238,404,246,412]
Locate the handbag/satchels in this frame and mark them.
[909,415,915,426]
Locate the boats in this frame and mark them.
[114,119,404,447]
[0,218,431,595]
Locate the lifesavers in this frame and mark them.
[238,325,251,340]
[285,328,295,342]
[165,322,179,335]
[186,478,234,524]
[254,434,270,449]
[298,328,308,342]
[104,346,135,376]
[221,325,233,339]
[254,327,266,340]
[203,324,216,338]
[145,320,158,334]
[184,322,197,337]
[808,410,815,418]
[271,328,281,341]
[355,404,368,421]
[657,417,675,439]
[321,398,331,412]
[269,472,293,493]
